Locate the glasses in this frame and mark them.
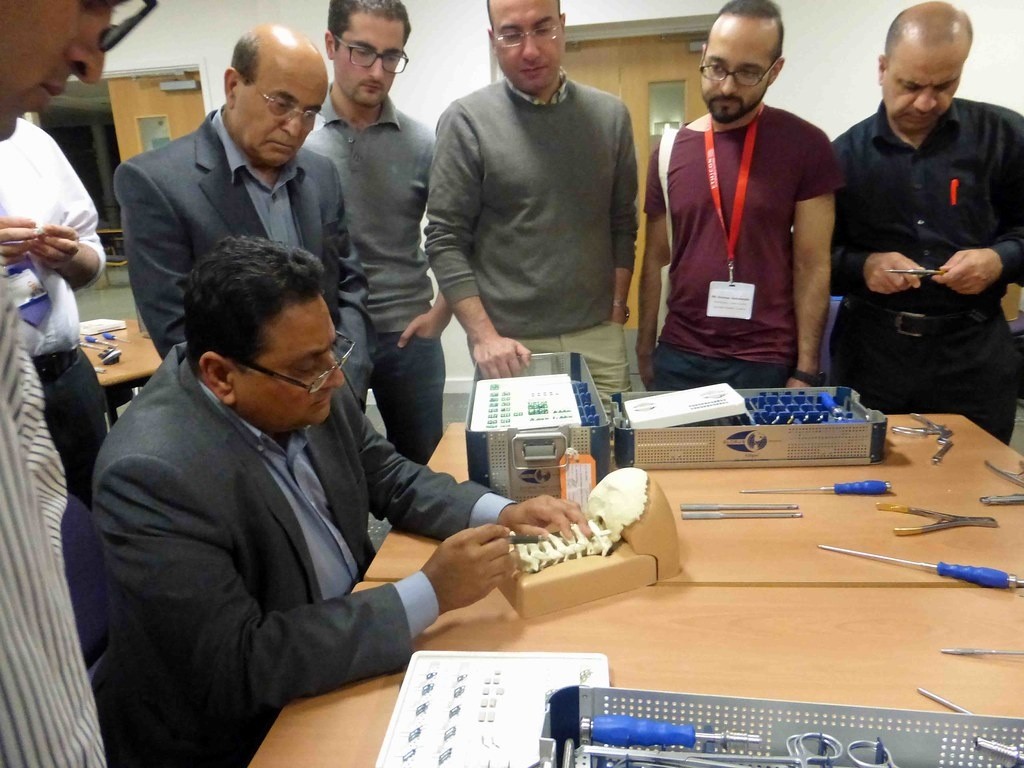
[226,329,356,393]
[698,45,781,87]
[244,75,326,131]
[494,15,562,48]
[98,0,156,54]
[334,35,409,74]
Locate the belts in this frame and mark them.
[842,293,999,337]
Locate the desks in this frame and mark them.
[362,412,1024,579]
[78,314,164,427]
[240,580,1024,768]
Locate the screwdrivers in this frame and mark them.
[589,719,763,747]
[103,333,131,343]
[816,544,1024,590]
[84,336,116,346]
[738,480,892,495]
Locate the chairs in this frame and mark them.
[59,495,108,680]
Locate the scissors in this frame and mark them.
[582,731,845,768]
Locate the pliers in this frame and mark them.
[876,503,998,536]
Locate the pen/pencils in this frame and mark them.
[950,178,959,205]
[504,534,543,544]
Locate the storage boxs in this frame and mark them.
[538,684,1024,768]
[465,349,609,506]
[610,385,888,471]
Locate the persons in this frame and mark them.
[823,0,1024,442]
[0,0,157,768]
[0,117,106,509]
[92,236,594,768]
[634,0,846,394]
[115,23,375,417]
[424,0,640,418]
[302,0,453,466]
